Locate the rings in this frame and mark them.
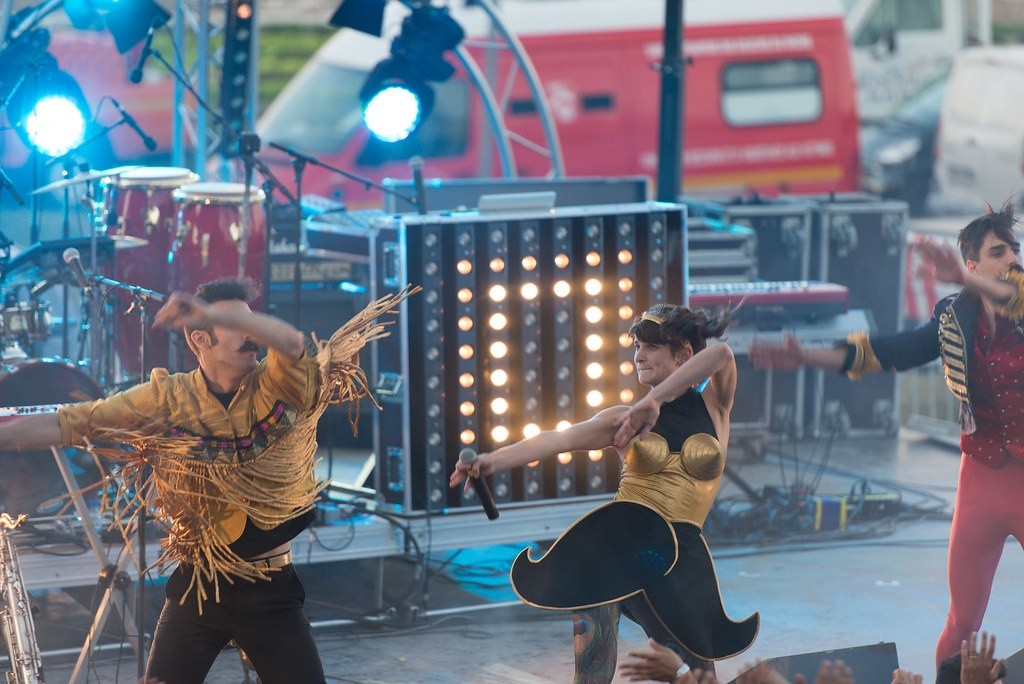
[970,656,977,658]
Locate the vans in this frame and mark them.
[188,0,1024,212]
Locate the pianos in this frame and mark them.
[689,277,847,324]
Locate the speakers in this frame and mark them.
[728,642,898,684]
[997,649,1024,684]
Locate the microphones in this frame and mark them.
[129,18,158,82]
[111,99,159,152]
[63,248,95,299]
[458,448,500,521]
[409,155,427,215]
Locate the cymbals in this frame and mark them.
[32,167,129,194]
[104,234,150,249]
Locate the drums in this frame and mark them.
[1,358,110,516]
[110,167,199,377]
[168,183,268,371]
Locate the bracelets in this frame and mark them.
[669,663,690,683]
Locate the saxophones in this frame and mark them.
[1,509,48,684]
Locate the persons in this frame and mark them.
[0,276,422,684]
[449,304,761,684]
[619,629,1009,684]
[750,193,1023,673]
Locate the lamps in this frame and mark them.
[327,0,385,37]
[7,70,91,159]
[360,60,435,144]
